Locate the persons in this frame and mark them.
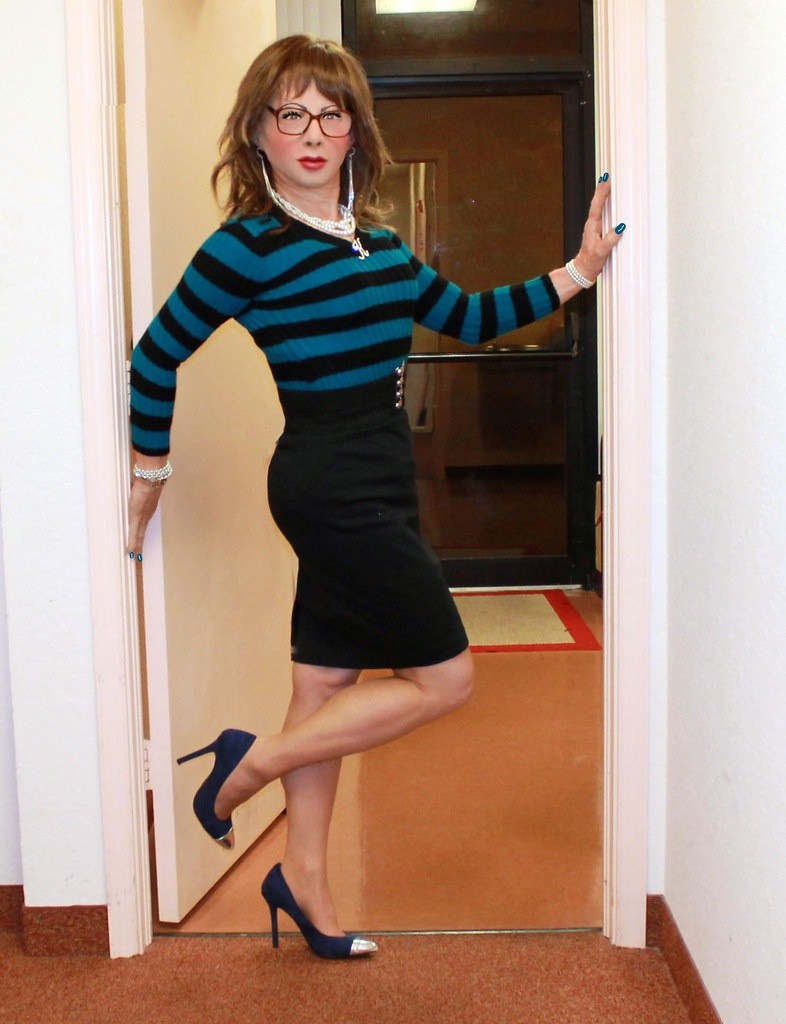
[126,34,625,960]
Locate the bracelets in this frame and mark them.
[132,461,172,482]
[565,259,593,290]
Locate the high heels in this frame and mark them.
[262,862,379,959]
[176,729,257,850]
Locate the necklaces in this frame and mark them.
[270,184,356,234]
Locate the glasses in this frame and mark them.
[264,102,356,139]
